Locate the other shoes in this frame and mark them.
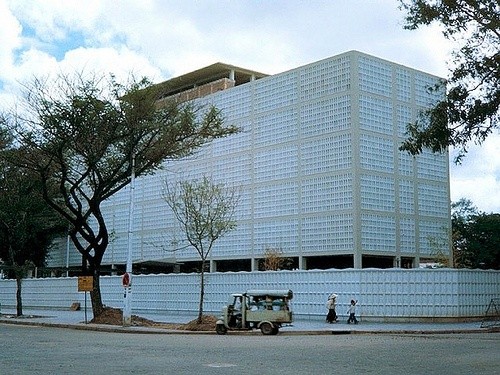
[330,321,333,324]
[347,320,351,324]
[355,321,359,324]
[335,320,339,322]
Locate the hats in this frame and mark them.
[329,294,337,299]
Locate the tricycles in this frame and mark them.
[216,290,293,335]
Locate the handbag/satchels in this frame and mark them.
[326,300,333,308]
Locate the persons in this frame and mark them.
[229,295,244,327]
[345,299,360,324]
[324,293,340,324]
